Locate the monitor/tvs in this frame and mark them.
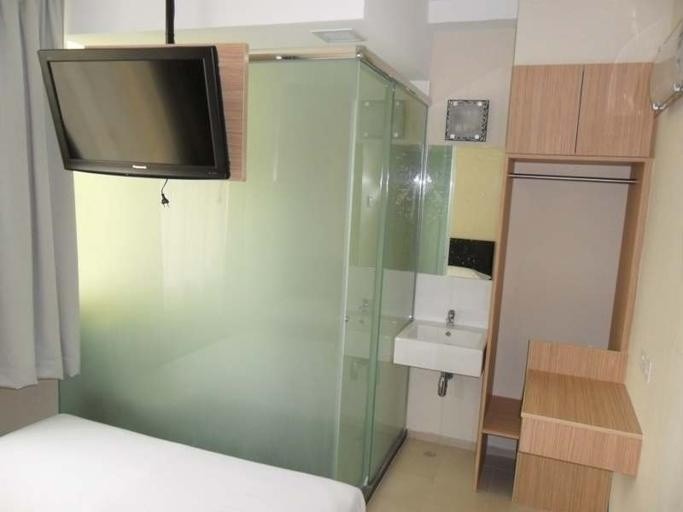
[37,45,231,180]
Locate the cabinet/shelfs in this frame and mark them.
[509,374,641,512]
[473,62,658,493]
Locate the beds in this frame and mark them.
[0,413,367,512]
